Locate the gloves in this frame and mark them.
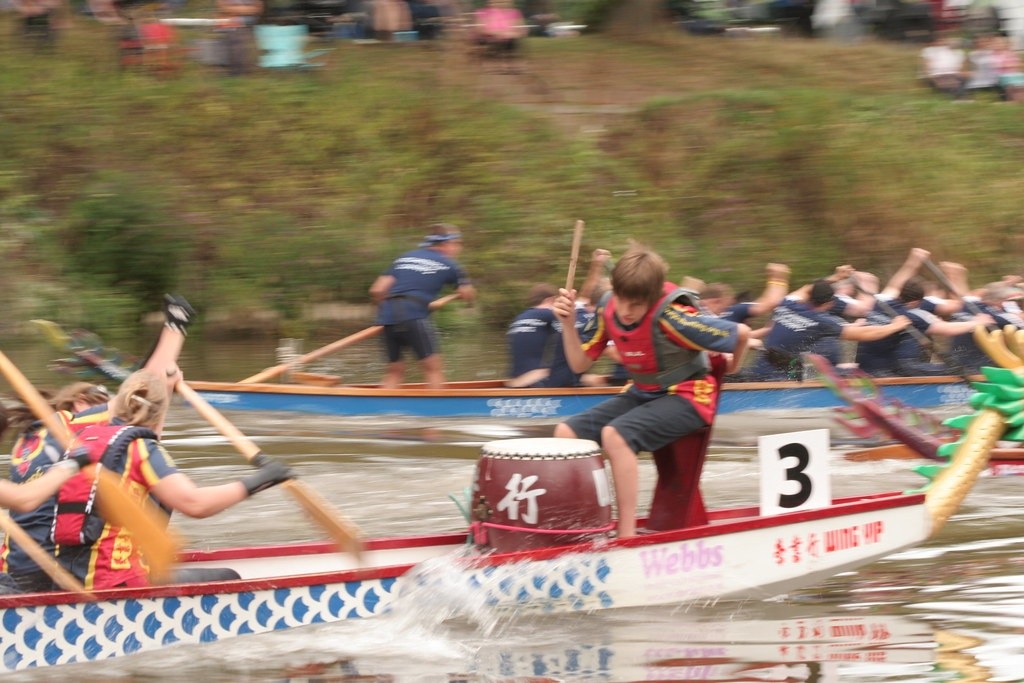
[67,445,93,471]
[240,459,295,497]
[164,290,197,338]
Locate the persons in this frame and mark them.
[474,0,528,74]
[368,222,472,389]
[773,0,1024,51]
[921,31,1024,102]
[554,250,751,537]
[114,20,172,77]
[0,293,299,595]
[505,262,1024,388]
[215,0,461,77]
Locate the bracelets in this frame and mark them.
[768,280,787,287]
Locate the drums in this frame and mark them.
[468,436,616,559]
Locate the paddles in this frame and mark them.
[1,510,86,599]
[1,353,186,571]
[235,288,458,385]
[847,271,976,390]
[923,259,1002,332]
[172,376,370,558]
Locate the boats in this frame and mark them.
[798,352,1024,481]
[0,363,1024,675]
[32,319,990,422]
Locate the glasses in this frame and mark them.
[77,384,110,398]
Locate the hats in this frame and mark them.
[697,284,725,300]
[897,279,926,303]
[1001,287,1024,300]
[809,281,835,307]
